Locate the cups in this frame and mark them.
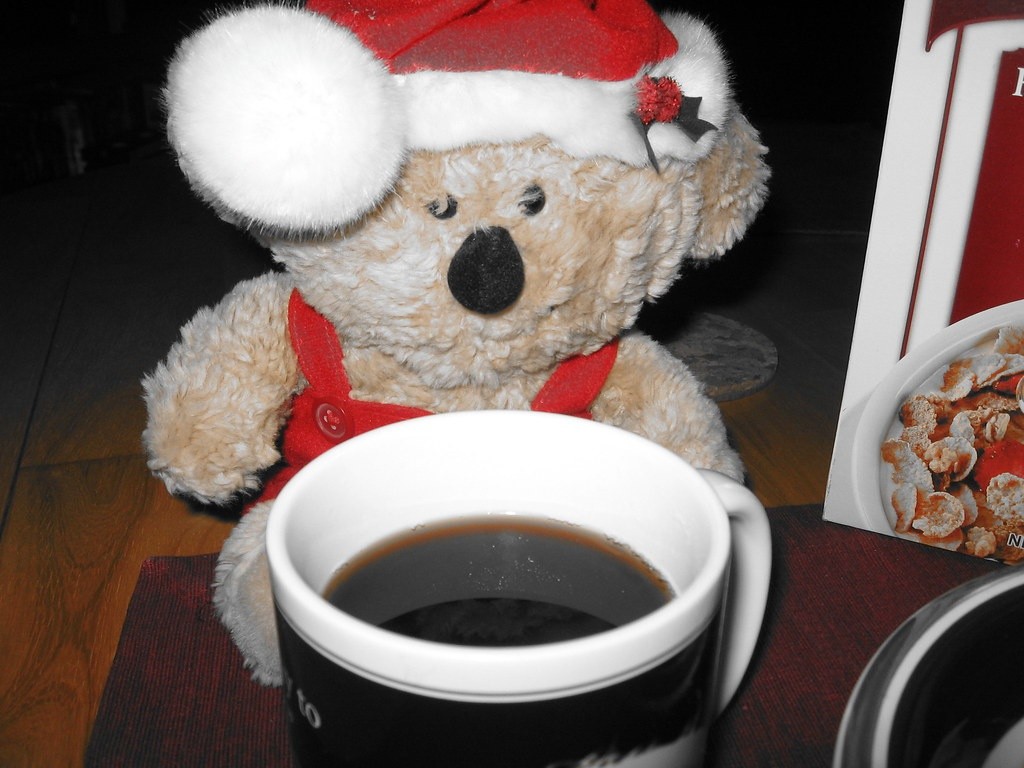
[264,410,773,768]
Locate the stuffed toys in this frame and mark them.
[139,1,775,507]
